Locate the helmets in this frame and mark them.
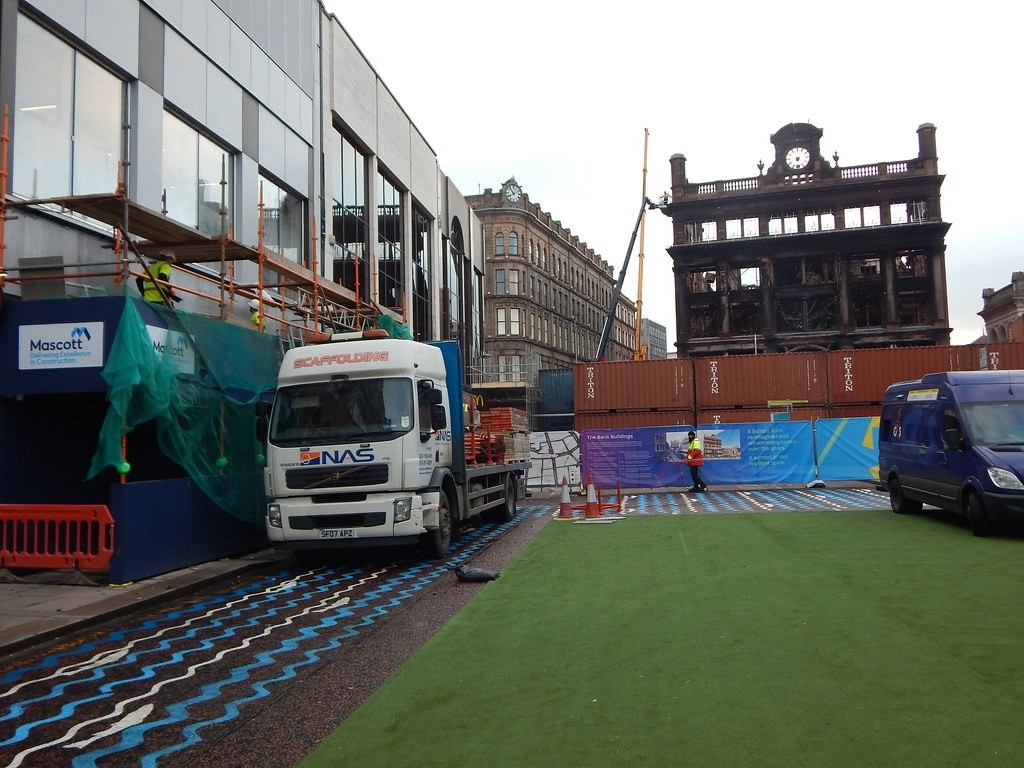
[248,299,260,310]
[158,248,176,262]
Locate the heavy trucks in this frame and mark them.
[262,328,533,568]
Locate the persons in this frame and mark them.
[247,298,267,332]
[685,431,708,492]
[136,250,176,307]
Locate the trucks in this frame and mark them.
[876,368,1024,539]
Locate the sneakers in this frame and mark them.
[689,485,708,493]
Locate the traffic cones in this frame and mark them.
[557,475,575,519]
[584,474,601,519]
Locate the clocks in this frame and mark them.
[505,183,523,204]
[784,144,814,172]
